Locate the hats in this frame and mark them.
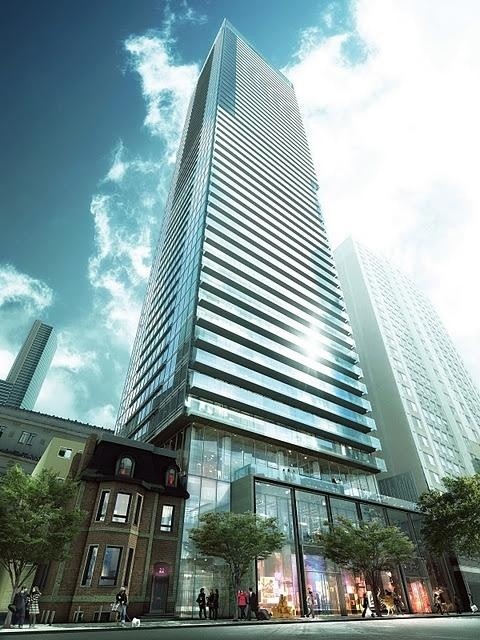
[120,587,125,592]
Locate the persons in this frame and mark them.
[114,585,129,628]
[193,586,476,618]
[10,585,29,627]
[27,587,42,627]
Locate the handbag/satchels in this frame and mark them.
[8,604,16,613]
[110,610,121,621]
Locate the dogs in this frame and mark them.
[132,617,141,627]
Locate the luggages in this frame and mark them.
[257,607,270,620]
